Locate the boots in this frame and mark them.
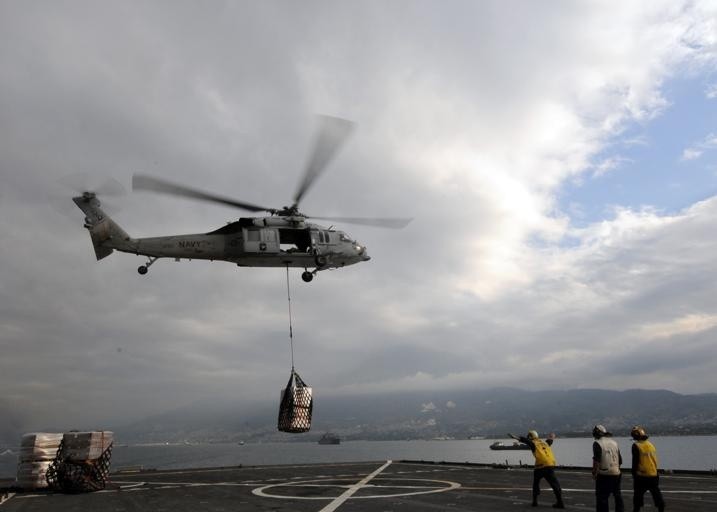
[532,493,539,508]
[552,493,563,509]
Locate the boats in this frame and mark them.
[489,440,535,450]
[318,432,340,445]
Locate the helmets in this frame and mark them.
[631,426,650,440]
[526,429,539,440]
[592,424,608,438]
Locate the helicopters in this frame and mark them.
[72,112,413,282]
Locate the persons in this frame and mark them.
[592,426,625,512]
[507,430,565,508]
[631,426,664,512]
